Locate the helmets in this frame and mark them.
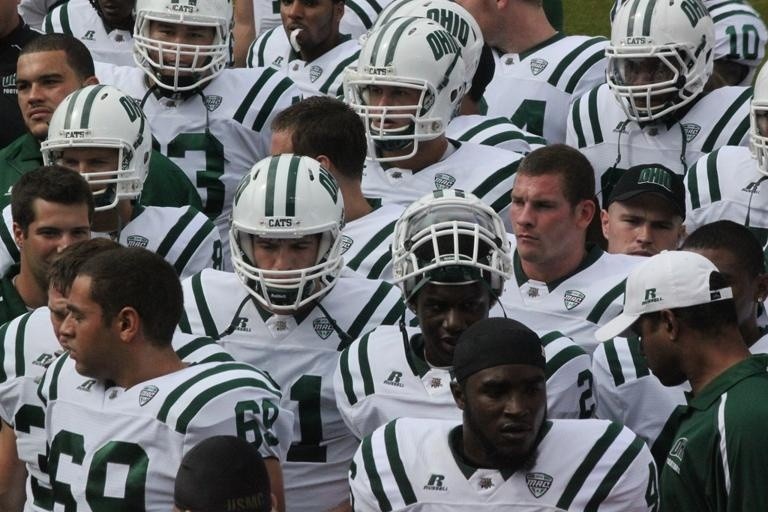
[41,84,152,213]
[229,155,347,310]
[603,0,714,124]
[344,15,466,160]
[391,189,511,309]
[748,58,768,176]
[132,0,235,99]
[371,0,485,100]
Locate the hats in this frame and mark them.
[595,250,733,342]
[607,164,686,223]
[172,435,269,512]
[452,317,546,384]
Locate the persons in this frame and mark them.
[0,0,768,512]
